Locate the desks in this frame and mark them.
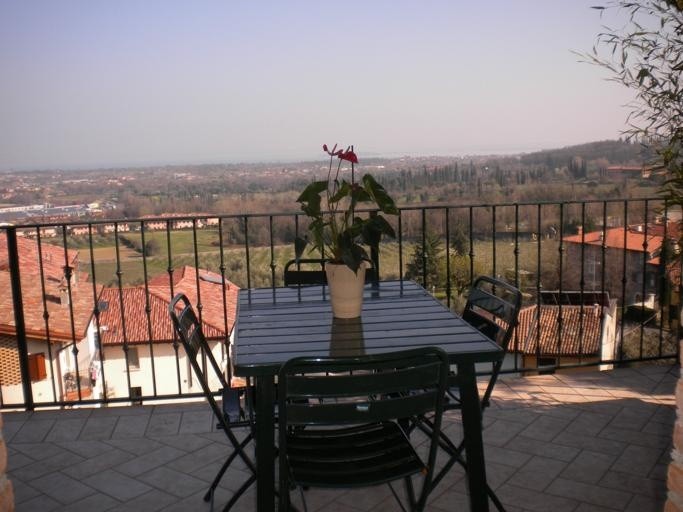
[232,278,503,512]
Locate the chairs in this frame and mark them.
[275,346,451,511]
[368,278,522,511]
[169,293,312,512]
[283,257,377,287]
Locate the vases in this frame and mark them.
[323,259,367,318]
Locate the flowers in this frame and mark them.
[294,143,401,278]
